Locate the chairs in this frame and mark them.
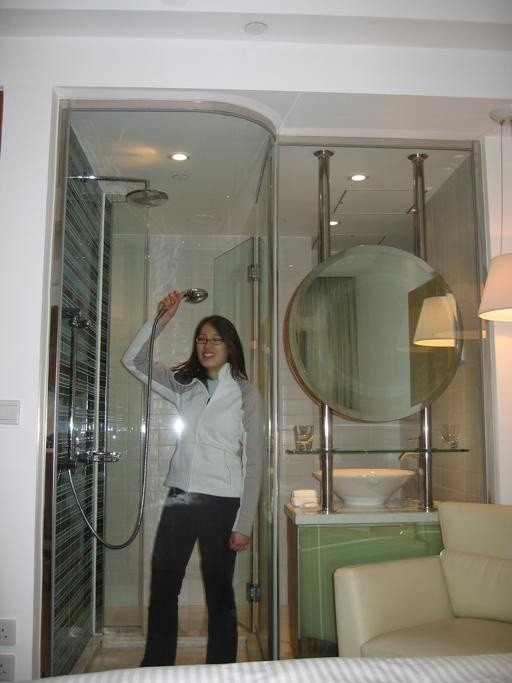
[332,500,512,659]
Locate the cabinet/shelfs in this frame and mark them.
[284,493,442,659]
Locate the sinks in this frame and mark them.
[310,467,416,511]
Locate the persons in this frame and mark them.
[121,289,266,668]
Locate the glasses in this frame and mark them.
[195,335,224,345]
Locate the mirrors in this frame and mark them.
[282,245,463,423]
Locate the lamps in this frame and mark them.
[412,294,458,348]
[476,253,512,325]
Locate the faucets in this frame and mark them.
[398,434,426,511]
